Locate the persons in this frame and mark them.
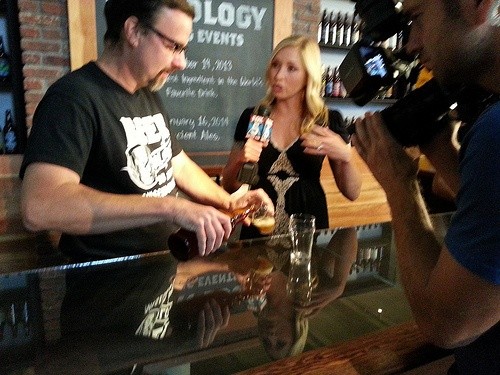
[20,0,275,264]
[33,228,358,375]
[352,1,499,375]
[223,35,363,240]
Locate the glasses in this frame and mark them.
[137,17,189,54]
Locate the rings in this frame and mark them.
[317,144,323,151]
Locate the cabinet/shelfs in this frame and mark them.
[317,40,398,105]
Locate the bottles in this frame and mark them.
[166,201,258,262]
[169,291,250,336]
[320,65,394,99]
[0,297,31,341]
[0,36,11,79]
[317,8,359,45]
[348,238,385,273]
[3,109,18,155]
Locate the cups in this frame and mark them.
[249,199,276,234]
[289,213,315,259]
[286,259,312,306]
[245,256,274,296]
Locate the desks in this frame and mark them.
[0,212,500,375]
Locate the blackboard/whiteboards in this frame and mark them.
[169,270,258,335]
[67,0,293,177]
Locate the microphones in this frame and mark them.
[247,293,269,321]
[237,104,274,185]
